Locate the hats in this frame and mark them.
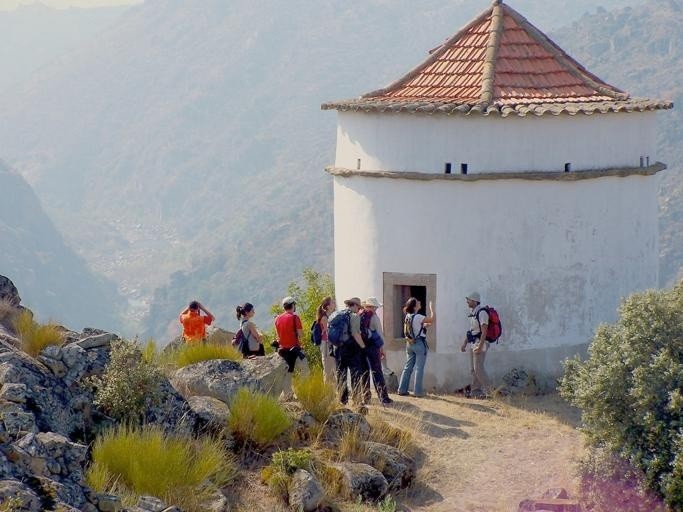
[344,297,364,310]
[466,291,480,302]
[363,298,383,307]
[282,296,296,304]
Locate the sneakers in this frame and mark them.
[380,398,392,403]
[398,392,409,396]
[466,392,482,398]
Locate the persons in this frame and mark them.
[274,296,311,377]
[316,296,395,409]
[396,297,435,397]
[178,300,216,344]
[460,291,492,398]
[235,302,265,359]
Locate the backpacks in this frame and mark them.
[232,320,251,356]
[404,316,423,343]
[328,312,352,346]
[357,309,376,342]
[310,320,322,344]
[477,307,502,343]
